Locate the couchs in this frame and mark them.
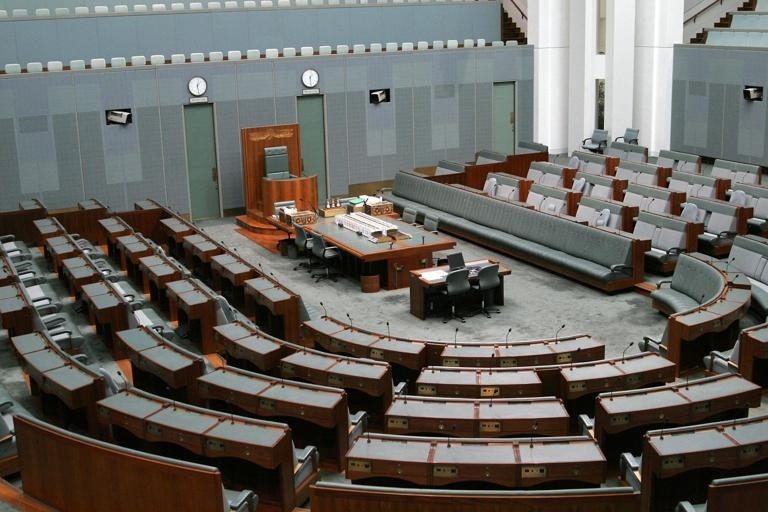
[377,169,644,295]
[637,253,752,359]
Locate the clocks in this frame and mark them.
[301,68,320,88]
[187,75,208,97]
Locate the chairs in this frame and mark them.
[583,128,611,154]
[436,270,471,323]
[614,128,641,144]
[265,145,297,178]
[473,265,502,319]
[310,231,345,283]
[396,208,418,225]
[417,214,440,234]
[292,222,323,273]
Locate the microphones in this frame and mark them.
[538,198,546,211]
[489,353,495,375]
[647,199,655,211]
[454,328,459,348]
[505,328,512,346]
[570,347,581,371]
[319,302,328,320]
[221,240,229,254]
[446,426,456,449]
[610,377,621,402]
[176,212,184,219]
[347,352,352,364]
[553,154,559,163]
[168,207,176,213]
[404,379,410,404]
[489,388,498,409]
[622,341,634,364]
[698,294,705,312]
[636,172,642,184]
[191,221,198,229]
[679,160,688,171]
[258,263,265,277]
[234,248,242,261]
[508,189,515,201]
[733,402,750,431]
[697,184,704,195]
[538,173,546,185]
[117,370,130,396]
[530,420,539,449]
[428,349,435,373]
[555,325,565,344]
[595,213,603,227]
[742,171,752,182]
[299,198,318,214]
[732,274,739,282]
[347,313,353,332]
[271,272,279,286]
[659,417,670,441]
[202,228,209,239]
[386,322,391,341]
[557,203,566,217]
[726,257,736,275]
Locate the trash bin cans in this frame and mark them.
[360,274,380,292]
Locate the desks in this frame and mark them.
[578,373,761,450]
[345,433,609,487]
[11,332,129,423]
[409,259,512,321]
[302,314,604,367]
[382,396,569,436]
[414,351,678,394]
[158,213,321,346]
[95,387,319,511]
[96,214,259,353]
[619,414,768,510]
[196,365,369,472]
[115,324,213,401]
[212,320,407,411]
[303,214,456,291]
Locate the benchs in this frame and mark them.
[0,235,32,261]
[608,142,649,162]
[61,254,119,297]
[517,141,547,153]
[45,233,96,272]
[727,233,768,321]
[481,172,533,201]
[434,160,466,174]
[622,181,686,216]
[77,197,107,210]
[80,281,174,342]
[4,256,46,285]
[576,195,639,233]
[656,149,701,173]
[680,195,754,259]
[710,159,762,185]
[134,198,162,209]
[571,170,628,200]
[632,210,705,273]
[18,277,63,313]
[668,171,732,201]
[676,473,767,511]
[526,160,576,189]
[701,323,768,378]
[18,197,45,211]
[310,481,641,510]
[12,414,256,511]
[472,150,507,165]
[569,151,620,177]
[615,160,672,187]
[30,305,84,348]
[526,182,581,216]
[729,182,767,224]
[32,217,66,236]
[246,275,321,338]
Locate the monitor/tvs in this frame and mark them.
[447,252,466,270]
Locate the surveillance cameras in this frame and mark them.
[371,90,386,103]
[107,110,132,124]
[743,88,761,100]
